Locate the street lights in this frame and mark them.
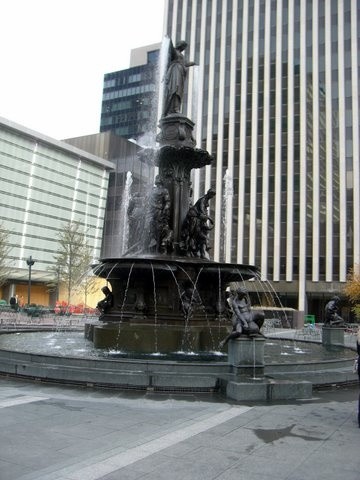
[27,256,35,305]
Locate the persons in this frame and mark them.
[128,176,218,260]
[163,37,196,115]
[178,288,198,319]
[218,285,265,349]
[94,284,117,317]
[10,293,24,310]
[356,330,360,428]
[323,295,345,327]
